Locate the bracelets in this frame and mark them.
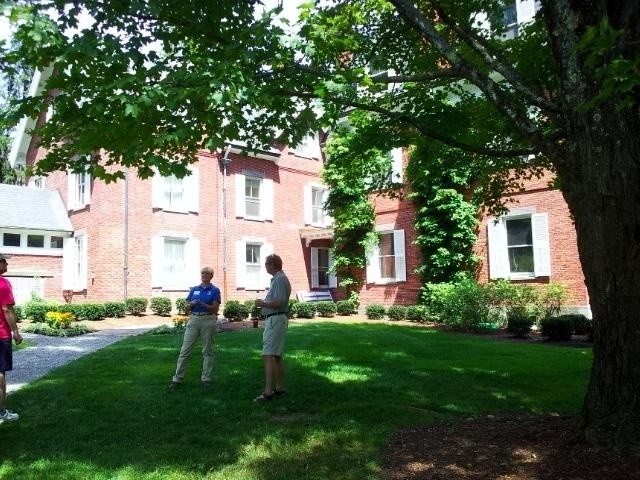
[263,301,267,307]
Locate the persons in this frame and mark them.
[0,253,25,426]
[252,254,293,402]
[168,266,221,392]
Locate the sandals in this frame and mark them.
[252,387,287,402]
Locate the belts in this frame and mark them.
[264,312,284,318]
[192,312,213,316]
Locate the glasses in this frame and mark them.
[1,259,6,263]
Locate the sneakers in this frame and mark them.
[202,381,211,386]
[0,410,19,425]
[170,381,180,386]
